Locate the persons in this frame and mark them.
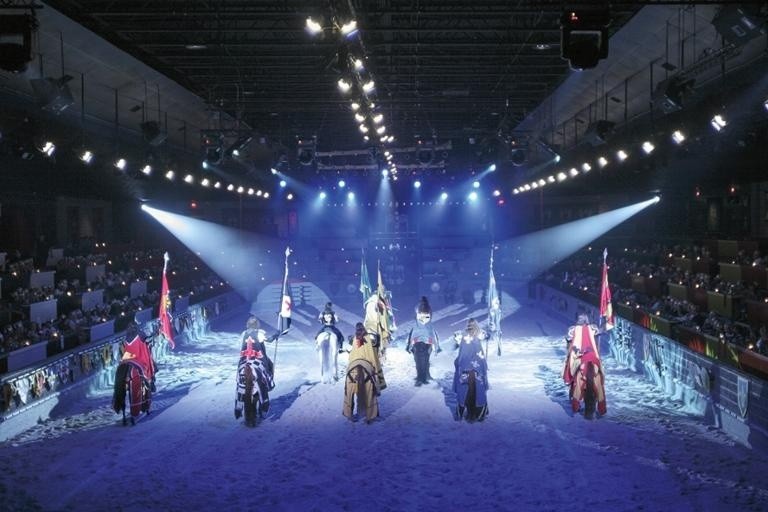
[539,226,767,358]
[0,237,247,409]
[238,315,281,371]
[448,317,490,368]
[404,296,443,356]
[347,319,380,359]
[313,299,346,354]
[563,308,605,386]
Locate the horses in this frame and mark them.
[234,356,275,427]
[111,340,152,427]
[343,334,381,424]
[563,336,606,420]
[315,331,341,384]
[454,343,490,423]
[411,342,433,387]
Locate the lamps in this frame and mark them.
[412,0,727,200]
[304,0,399,181]
[36,35,304,199]
[316,179,358,199]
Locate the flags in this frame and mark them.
[358,250,373,309]
[160,275,176,349]
[488,268,501,356]
[277,254,293,337]
[377,270,398,332]
